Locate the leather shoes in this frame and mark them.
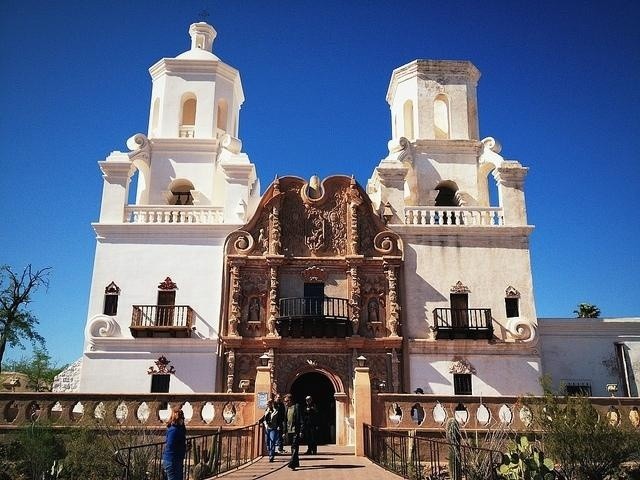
[269,449,317,469]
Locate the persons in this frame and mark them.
[255,399,282,462]
[161,407,186,480]
[272,395,288,451]
[413,386,425,422]
[300,395,320,455]
[280,393,305,470]
